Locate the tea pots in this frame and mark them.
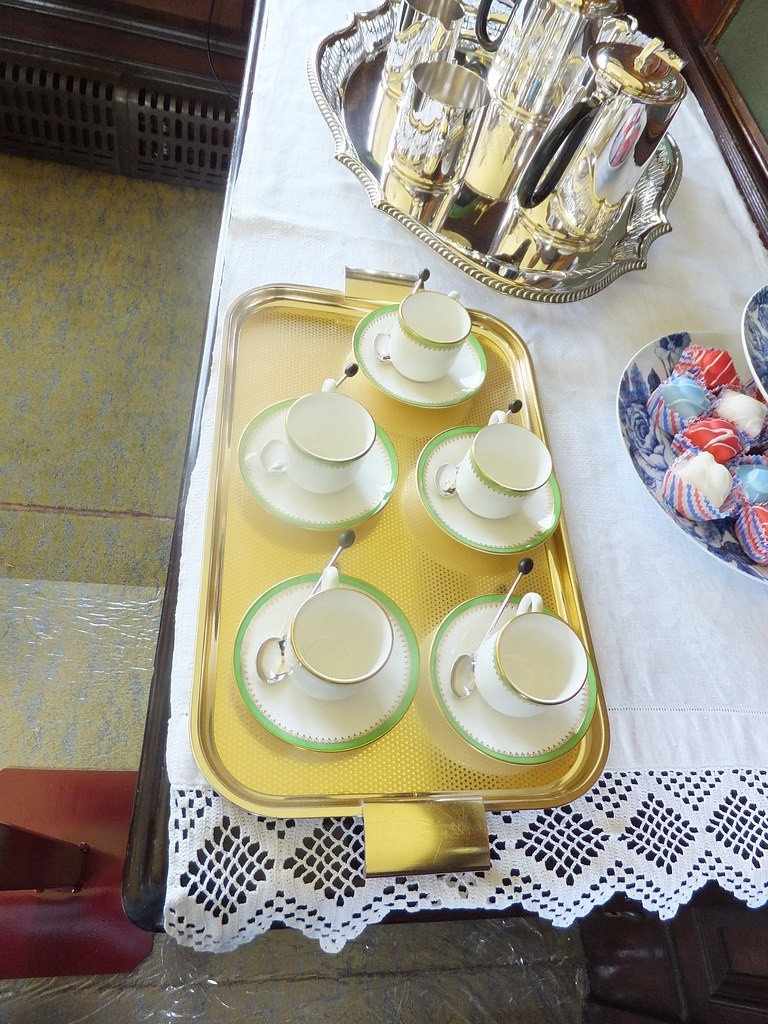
[474,0,624,121]
[514,37,692,250]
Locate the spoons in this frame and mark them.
[434,399,523,499]
[259,363,359,477]
[450,557,538,700]
[373,267,430,364]
[255,533,359,685]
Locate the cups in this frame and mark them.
[381,0,468,107]
[455,410,552,519]
[389,290,472,382]
[282,377,377,494]
[279,562,394,701]
[389,58,492,193]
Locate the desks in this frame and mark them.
[119,0,768,933]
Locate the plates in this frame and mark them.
[351,303,487,409]
[475,591,590,718]
[428,592,599,764]
[234,568,420,754]
[616,331,768,585]
[414,423,561,556]
[189,284,612,878]
[741,281,768,403]
[237,395,398,531]
[304,0,684,303]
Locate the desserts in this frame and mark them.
[646,346,768,569]
